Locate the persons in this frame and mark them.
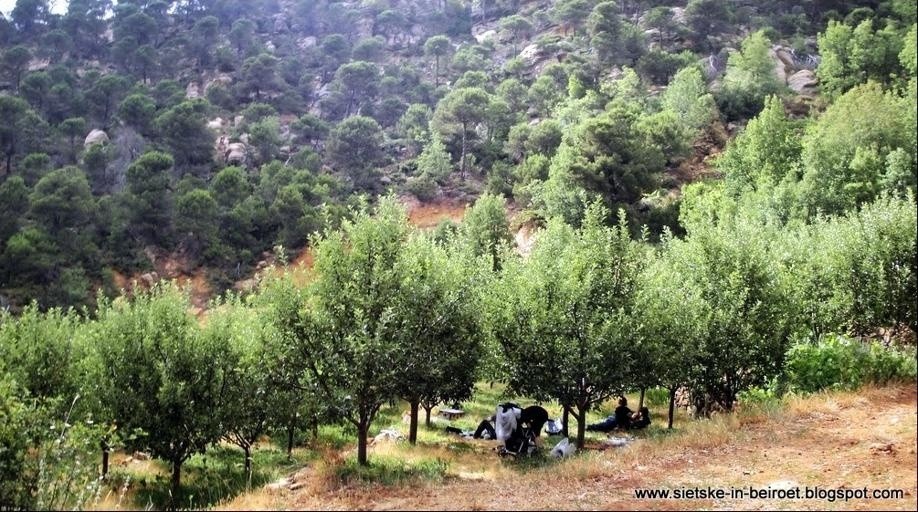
[473,391,650,440]
